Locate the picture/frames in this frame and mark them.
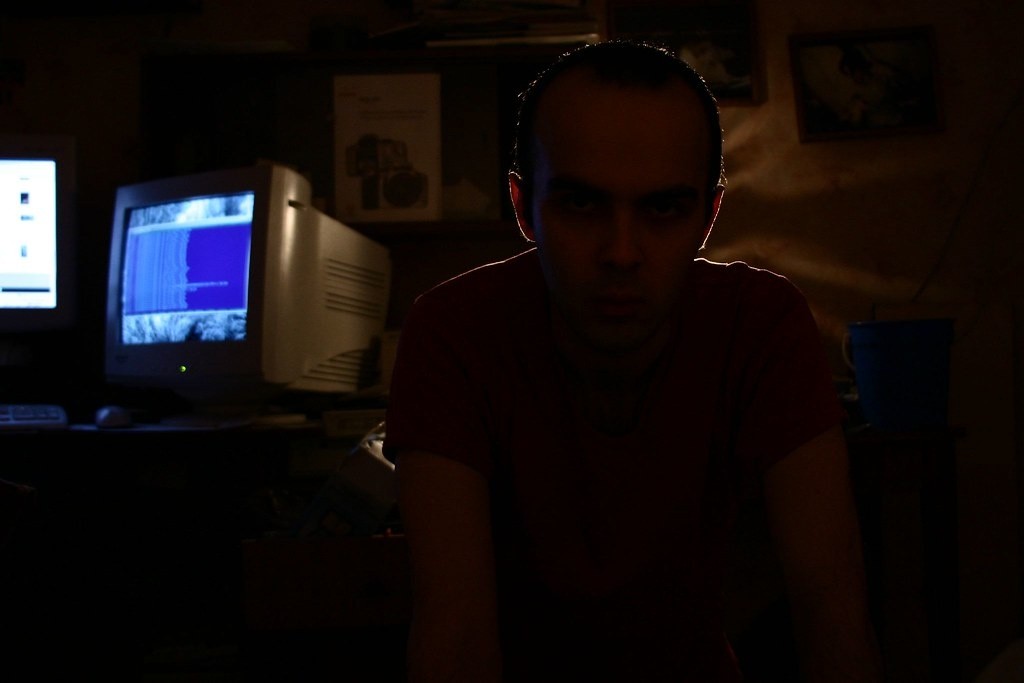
[606,0,766,108]
[785,21,949,143]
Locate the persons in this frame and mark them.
[382,43,881,683]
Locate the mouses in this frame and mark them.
[97,405,132,427]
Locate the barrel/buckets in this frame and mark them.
[840,319,953,417]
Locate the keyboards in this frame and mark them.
[0,403,69,433]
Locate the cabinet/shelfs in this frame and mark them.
[155,42,576,245]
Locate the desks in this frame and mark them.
[843,429,970,683]
[0,413,319,468]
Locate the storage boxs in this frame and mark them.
[244,533,422,632]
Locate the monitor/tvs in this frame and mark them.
[0,132,78,328]
[104,159,392,426]
